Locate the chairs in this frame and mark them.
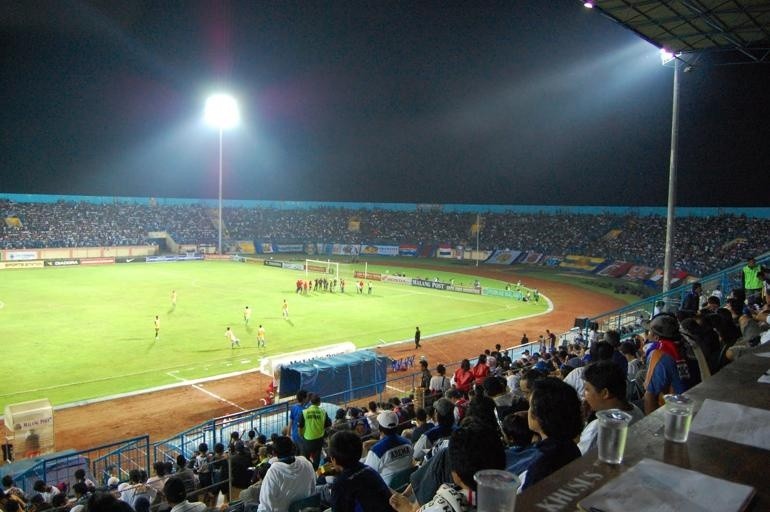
[244,288,770,512]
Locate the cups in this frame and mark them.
[662,393,695,444]
[594,407,634,466]
[472,468,519,512]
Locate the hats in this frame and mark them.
[642,315,680,337]
[377,410,399,429]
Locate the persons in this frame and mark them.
[282,298,289,319]
[415,326,422,350]
[577,211,768,272]
[154,315,162,339]
[224,327,241,349]
[577,255,770,456]
[243,306,250,325]
[256,325,266,347]
[382,270,540,305]
[171,291,177,306]
[295,276,374,297]
[0,200,575,257]
[1,328,576,511]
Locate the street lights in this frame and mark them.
[198,92,243,254]
[659,45,684,301]
